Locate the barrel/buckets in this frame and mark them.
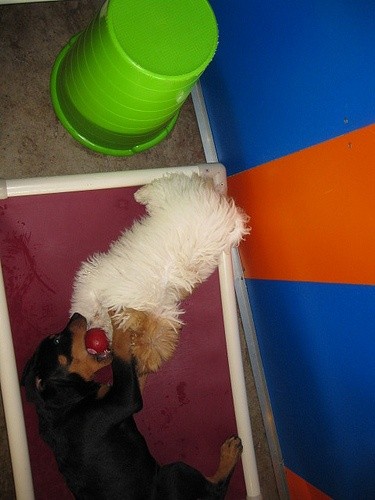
[50,0,218,156]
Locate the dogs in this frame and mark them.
[69,170,252,375]
[21,306,243,500]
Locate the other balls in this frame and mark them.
[85,328,110,355]
